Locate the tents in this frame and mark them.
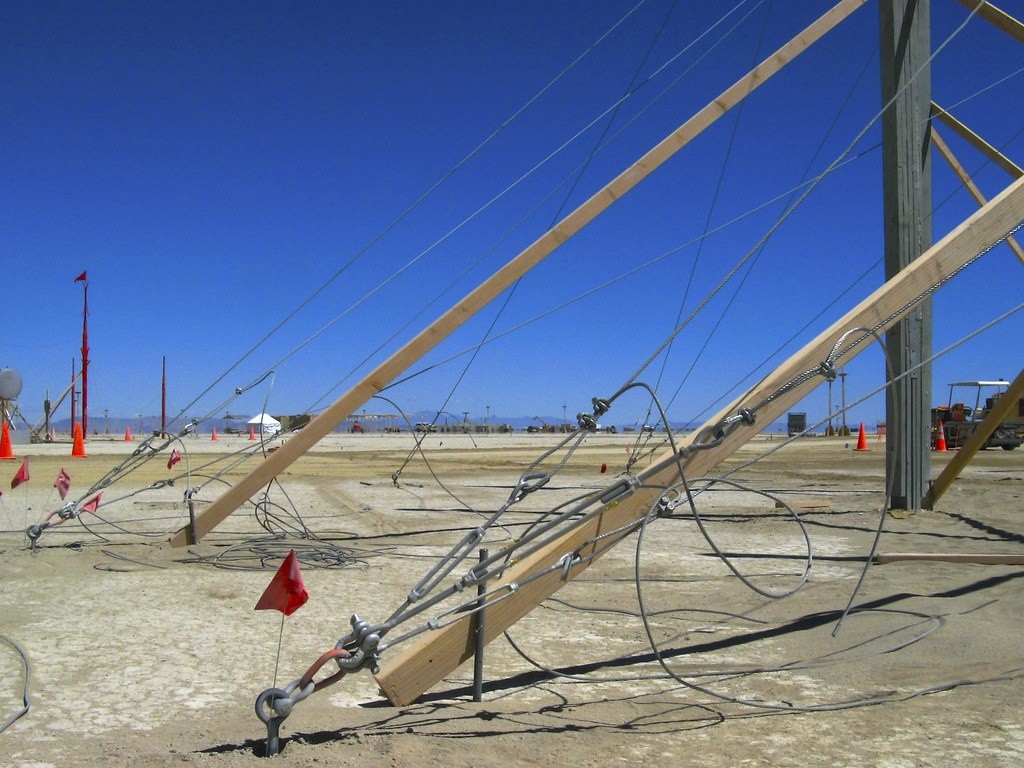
[247,413,282,436]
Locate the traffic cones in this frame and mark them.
[209,426,219,441]
[49,427,56,441]
[124,426,133,442]
[248,426,256,440]
[0,422,17,459]
[934,421,947,452]
[853,422,870,451]
[65,422,88,458]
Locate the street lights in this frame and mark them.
[562,400,567,425]
[486,405,490,418]
[463,412,469,429]
[75,384,81,421]
[826,378,837,436]
[838,367,848,436]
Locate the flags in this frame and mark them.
[251,550,309,616]
[0,447,181,513]
[74,270,86,283]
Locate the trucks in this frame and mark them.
[787,412,807,437]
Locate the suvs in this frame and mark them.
[416,422,437,433]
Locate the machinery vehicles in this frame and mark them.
[533,416,554,433]
[932,378,1024,450]
[351,420,364,434]
